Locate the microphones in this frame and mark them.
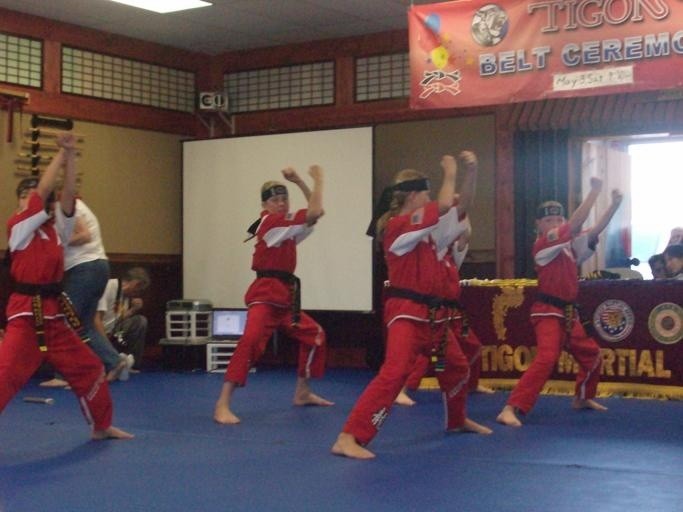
[625,258,640,267]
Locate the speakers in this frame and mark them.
[199,91,229,111]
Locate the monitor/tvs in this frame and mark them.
[212,308,249,339]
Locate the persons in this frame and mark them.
[95,267,149,373]
[329,149,492,458]
[495,176,622,427]
[40,187,126,388]
[1,132,137,441]
[663,245,682,280]
[393,193,495,406]
[666,227,682,247]
[212,163,334,424]
[647,253,667,279]
